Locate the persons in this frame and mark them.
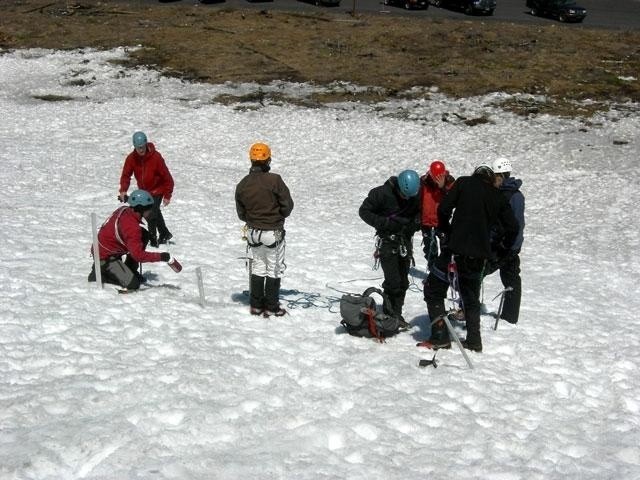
[116,131,175,247]
[234,142,294,318]
[358,169,423,327]
[88,189,170,291]
[415,166,520,353]
[420,161,456,271]
[445,157,526,324]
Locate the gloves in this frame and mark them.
[117,195,129,203]
[161,252,170,262]
[388,219,402,233]
[495,242,505,256]
[437,222,449,232]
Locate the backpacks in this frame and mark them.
[339,287,400,344]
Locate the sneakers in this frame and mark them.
[158,233,173,244]
[264,308,286,318]
[250,308,264,315]
[417,340,451,350]
[461,340,482,352]
[449,309,465,320]
[151,236,158,247]
[88,262,96,281]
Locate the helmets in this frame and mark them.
[249,143,271,160]
[492,158,513,174]
[474,160,493,177]
[397,170,420,198]
[430,161,445,176]
[127,190,155,208]
[132,131,147,147]
